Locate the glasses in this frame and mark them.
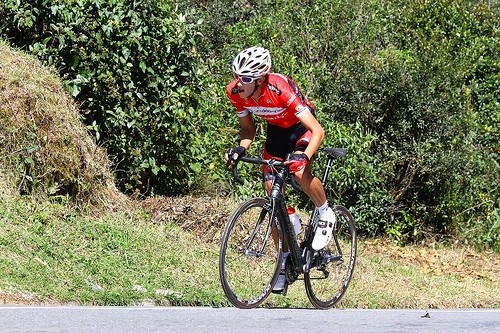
[232,73,259,83]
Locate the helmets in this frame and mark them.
[231,44,272,78]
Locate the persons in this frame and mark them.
[221,45,338,294]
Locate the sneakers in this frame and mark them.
[271,254,288,293]
[311,207,337,251]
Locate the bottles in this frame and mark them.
[287,205,302,235]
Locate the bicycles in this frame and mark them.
[219,148,357,310]
